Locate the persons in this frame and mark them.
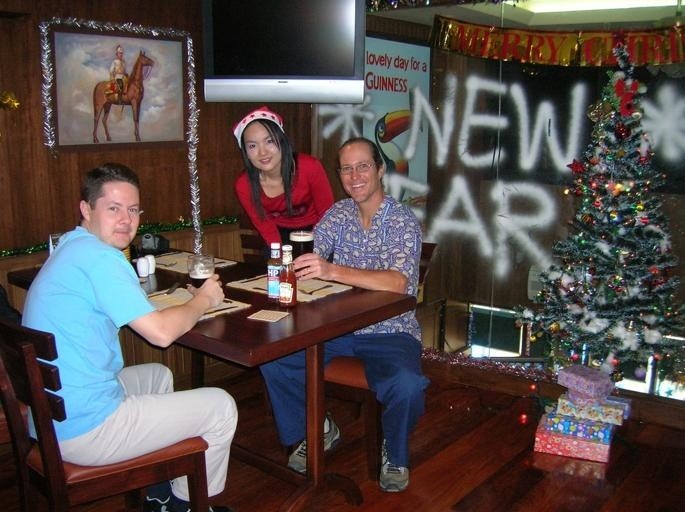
[20,161,239,511]
[230,105,334,267]
[109,45,130,106]
[259,137,429,493]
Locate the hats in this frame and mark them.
[232,105,286,149]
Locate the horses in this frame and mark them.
[92,50,155,143]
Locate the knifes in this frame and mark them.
[241,274,267,284]
[200,306,238,315]
[154,252,181,259]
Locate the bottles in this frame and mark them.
[277,245,298,307]
[266,242,280,303]
[136,254,155,278]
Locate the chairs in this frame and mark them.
[1,284,216,512]
[236,217,438,482]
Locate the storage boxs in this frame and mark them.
[533,365,632,464]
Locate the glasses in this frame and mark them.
[337,162,380,176]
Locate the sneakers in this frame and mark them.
[287,413,342,473]
[379,440,411,492]
[144,493,234,511]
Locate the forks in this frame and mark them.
[297,284,333,295]
[155,260,177,267]
[146,281,179,299]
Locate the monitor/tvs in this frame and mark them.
[200,0,367,104]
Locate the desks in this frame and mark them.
[6,249,417,512]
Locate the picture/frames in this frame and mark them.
[46,23,192,153]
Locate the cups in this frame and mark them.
[187,255,216,289]
[289,231,315,271]
